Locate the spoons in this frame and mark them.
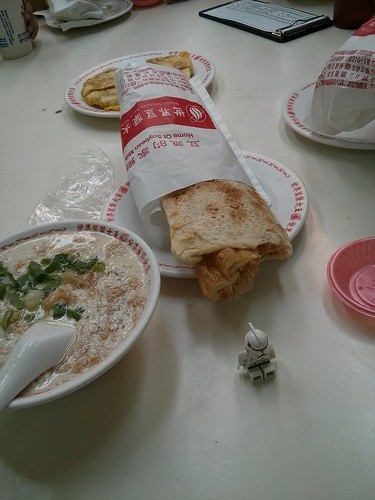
[0,322,75,408]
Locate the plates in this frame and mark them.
[102,151,308,279]
[45,0,133,28]
[281,79,374,149]
[65,48,215,118]
[326,237,375,318]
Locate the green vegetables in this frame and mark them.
[0,251,106,331]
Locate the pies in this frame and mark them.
[82,51,194,111]
[161,179,293,302]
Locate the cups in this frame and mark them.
[0,0,33,60]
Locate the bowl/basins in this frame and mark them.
[0,219,160,408]
